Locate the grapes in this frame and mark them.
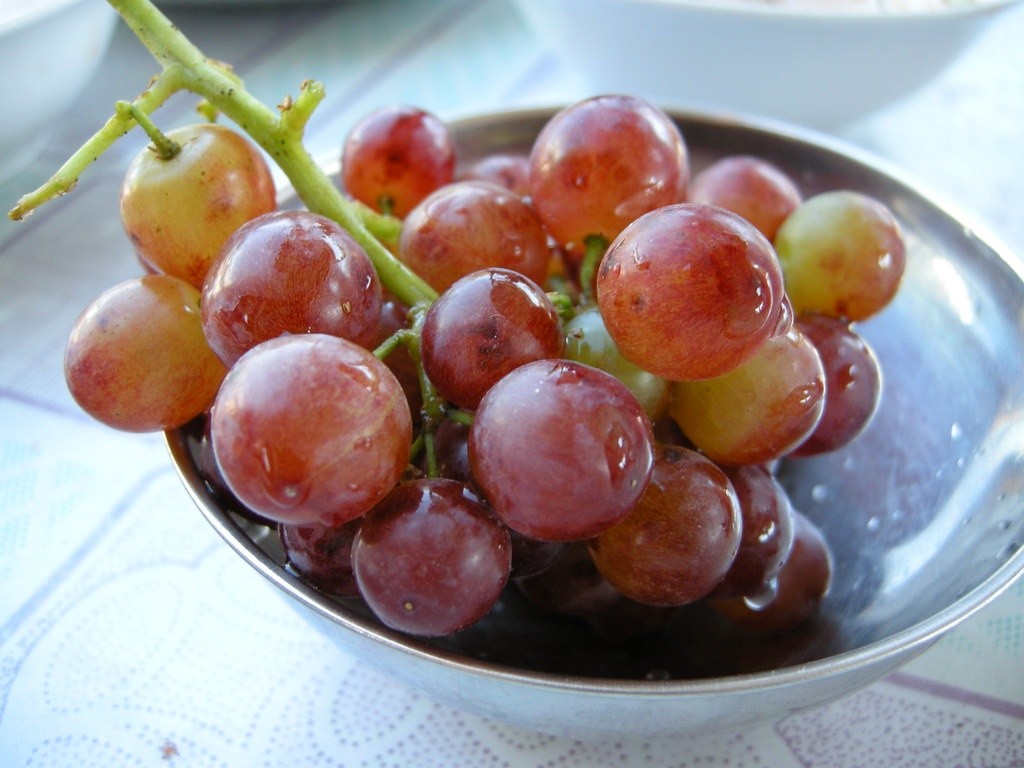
[59,94,910,642]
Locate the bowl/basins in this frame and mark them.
[507,0,1023,132]
[161,98,1024,735]
[0,0,120,186]
